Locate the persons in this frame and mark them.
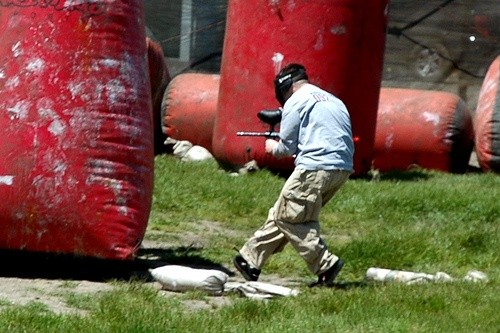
[233,60,354,285]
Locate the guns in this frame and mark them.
[236,109,283,139]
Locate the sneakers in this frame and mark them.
[234,255,261,281]
[317,259,344,285]
